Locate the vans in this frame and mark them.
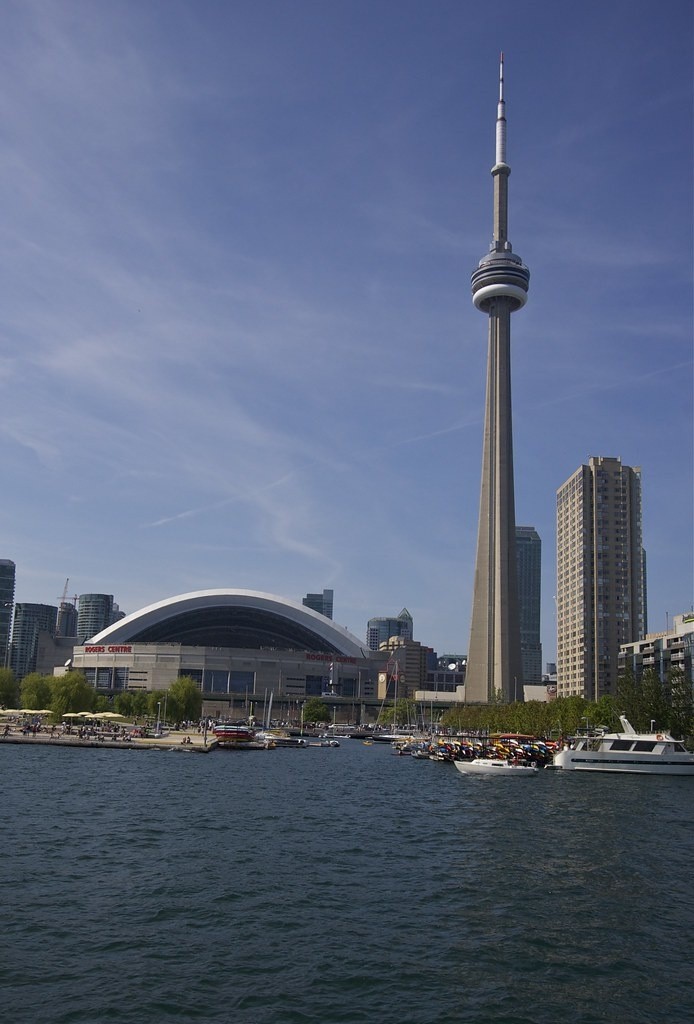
[321,692,337,697]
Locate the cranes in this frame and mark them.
[58,594,79,637]
[57,577,70,636]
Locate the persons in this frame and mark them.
[0,710,218,745]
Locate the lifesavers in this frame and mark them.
[655,735,664,741]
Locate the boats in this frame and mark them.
[454,758,538,775]
[213,724,559,767]
[552,715,694,775]
